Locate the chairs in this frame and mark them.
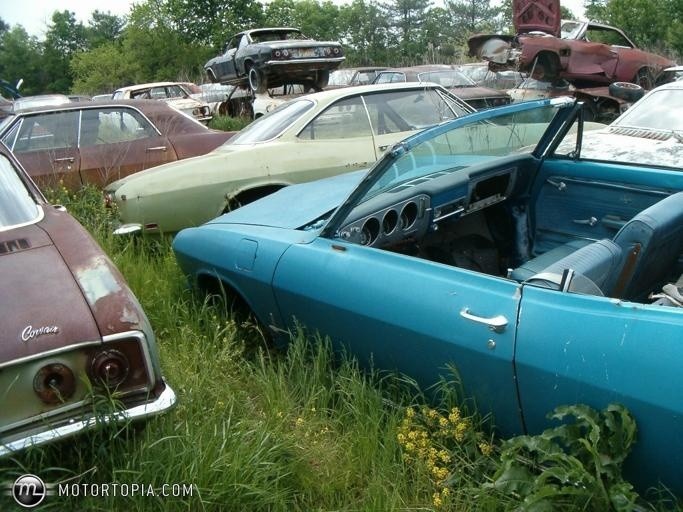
[506,190,681,303]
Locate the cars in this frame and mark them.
[1,1,683,507]
